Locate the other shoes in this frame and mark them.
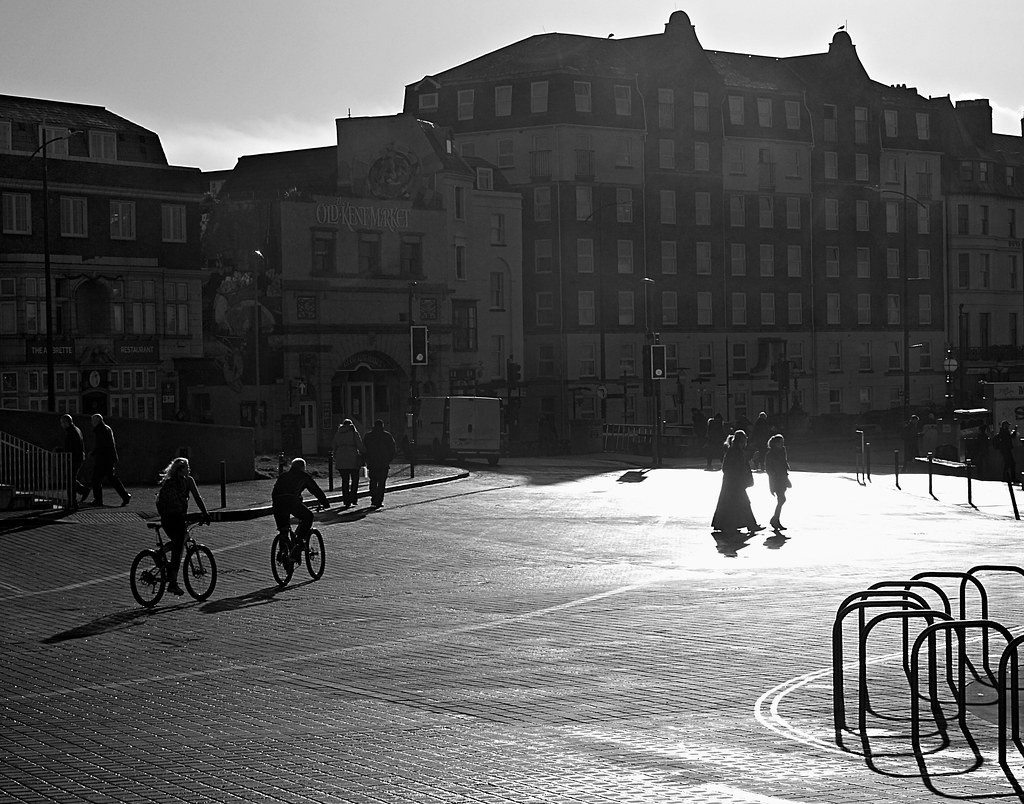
[167,584,184,597]
[349,498,358,505]
[121,494,132,508]
[747,524,766,531]
[80,486,92,501]
[376,504,383,507]
[371,502,376,505]
[772,522,786,530]
[90,499,102,506]
[345,503,350,507]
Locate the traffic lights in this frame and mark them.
[650,344,668,379]
[410,325,429,366]
[507,357,522,390]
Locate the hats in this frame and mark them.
[1002,420,1012,426]
[910,415,919,423]
[734,430,749,440]
[759,412,768,417]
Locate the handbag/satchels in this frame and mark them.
[786,478,791,487]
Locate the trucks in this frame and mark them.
[399,395,503,467]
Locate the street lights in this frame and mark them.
[252,248,265,424]
[642,276,663,468]
[862,164,929,420]
[19,120,87,440]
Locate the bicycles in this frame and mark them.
[130,517,219,609]
[270,503,328,585]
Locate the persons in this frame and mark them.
[53,413,91,509]
[751,412,771,471]
[154,457,211,597]
[710,429,766,534]
[907,415,923,463]
[704,412,725,466]
[997,419,1022,485]
[975,424,995,481]
[764,433,792,531]
[89,414,132,507]
[691,406,708,439]
[361,419,396,509]
[332,418,364,507]
[272,456,329,565]
